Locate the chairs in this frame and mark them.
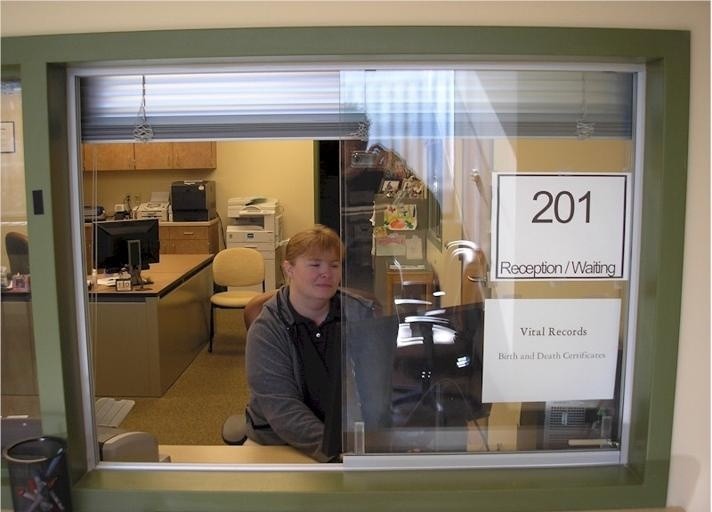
[208,248,265,353]
[5,232,29,275]
[223,286,382,445]
[99,430,154,462]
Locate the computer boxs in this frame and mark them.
[520,351,622,450]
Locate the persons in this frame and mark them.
[243,223,384,462]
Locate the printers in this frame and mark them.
[226,197,283,243]
[84,206,106,221]
[172,180,216,222]
[137,192,171,221]
[94,398,172,463]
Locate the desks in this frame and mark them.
[386,260,433,316]
[158,445,342,465]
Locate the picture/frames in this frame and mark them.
[1,122,15,154]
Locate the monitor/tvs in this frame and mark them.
[322,302,491,459]
[92,219,159,286]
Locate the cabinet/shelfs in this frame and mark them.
[160,222,220,254]
[83,141,217,172]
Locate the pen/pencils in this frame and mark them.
[17,448,65,512]
